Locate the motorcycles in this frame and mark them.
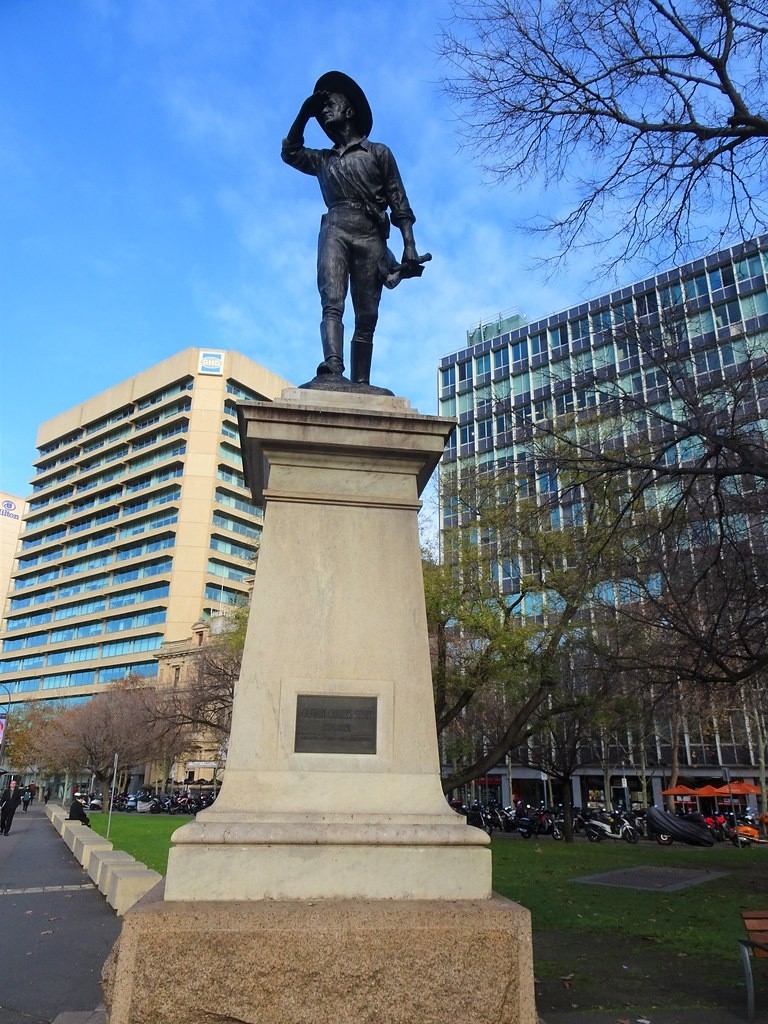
[71,777,223,818]
[448,790,768,848]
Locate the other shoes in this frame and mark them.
[4,832,8,836]
[0,830,3,835]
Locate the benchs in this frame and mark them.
[737,911,768,1024]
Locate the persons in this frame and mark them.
[18,783,25,804]
[282,91,421,385]
[30,781,36,805]
[0,781,20,836]
[21,786,32,812]
[44,793,50,804]
[70,795,89,825]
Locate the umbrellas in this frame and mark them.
[178,778,195,792]
[152,777,178,791]
[195,778,207,794]
[208,779,222,785]
